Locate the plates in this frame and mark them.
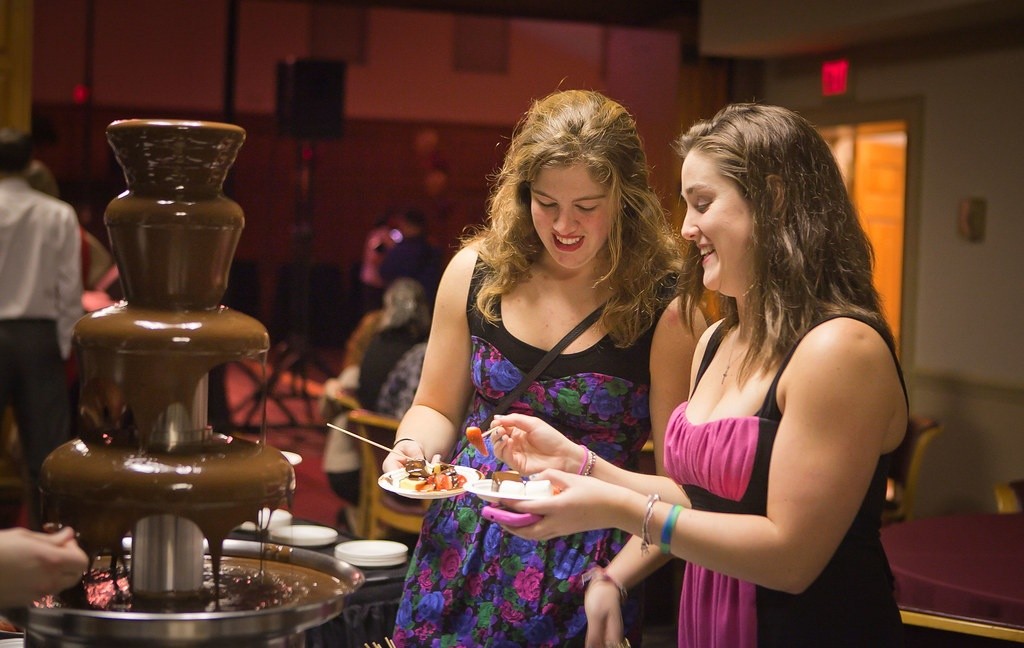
[279,450,303,466]
[239,508,409,567]
[378,463,486,500]
[0,629,24,648]
[463,479,539,502]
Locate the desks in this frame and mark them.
[878,512,1024,648]
[95,517,413,648]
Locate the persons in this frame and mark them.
[379,90,708,648]
[0,119,84,475]
[0,526,89,621]
[581,101,915,648]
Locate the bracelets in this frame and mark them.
[578,445,589,476]
[660,505,685,556]
[579,566,628,603]
[393,437,428,463]
[586,452,596,478]
[639,493,661,558]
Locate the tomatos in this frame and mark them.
[466,426,488,457]
[415,474,466,493]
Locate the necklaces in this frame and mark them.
[721,327,748,382]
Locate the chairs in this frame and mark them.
[880,413,944,526]
[347,407,433,557]
[320,378,387,542]
